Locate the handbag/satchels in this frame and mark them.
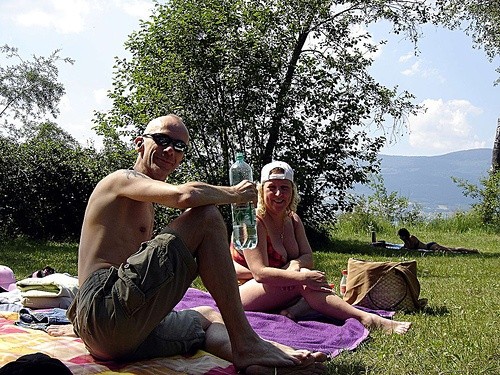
[343,257,418,312]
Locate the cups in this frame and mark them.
[372,232,376,242]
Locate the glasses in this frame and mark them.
[140,133,189,153]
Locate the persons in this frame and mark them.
[398,229,480,254]
[65,114,326,375]
[229,161,412,335]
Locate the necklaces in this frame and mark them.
[265,211,285,243]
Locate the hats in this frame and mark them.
[261,161,293,183]
[0,265,16,292]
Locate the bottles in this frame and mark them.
[329,284,335,292]
[340,270,347,296]
[229,153,258,250]
